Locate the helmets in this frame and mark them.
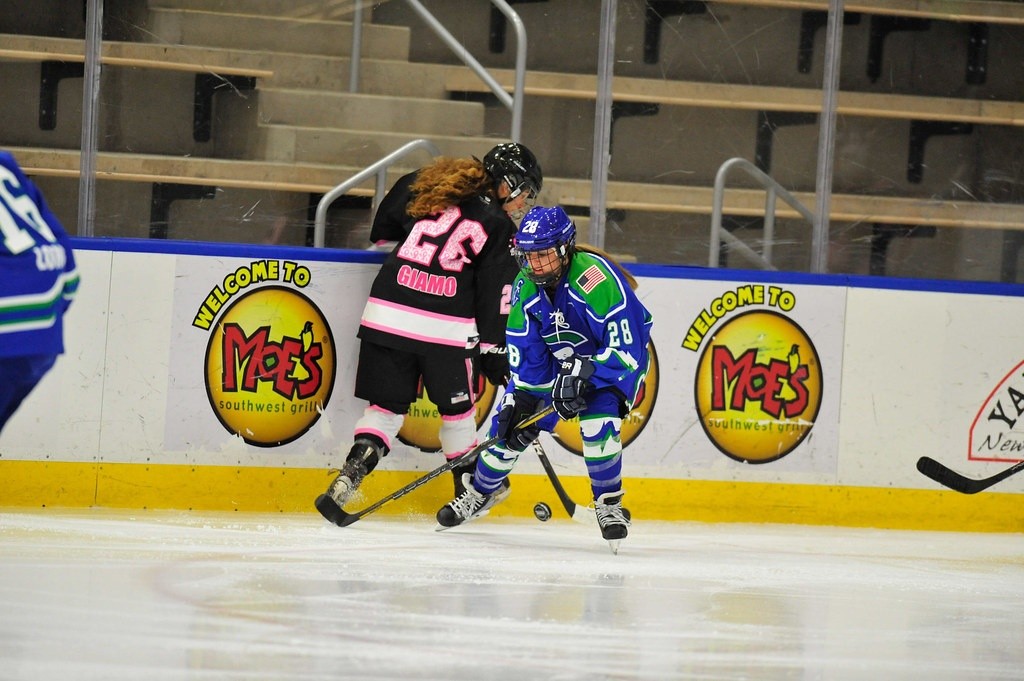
[510,206,576,286]
[483,143,543,207]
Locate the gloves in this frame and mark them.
[498,390,545,452]
[551,354,596,421]
[477,345,509,386]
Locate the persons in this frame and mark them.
[0,152,81,435]
[327,142,544,521]
[435,204,654,555]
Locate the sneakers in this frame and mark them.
[594,491,631,556]
[451,453,509,517]
[325,457,368,508]
[435,471,510,531]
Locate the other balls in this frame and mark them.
[534,502,552,522]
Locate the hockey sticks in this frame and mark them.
[916,456,1022,495]
[312,380,596,528]
[501,375,631,526]
